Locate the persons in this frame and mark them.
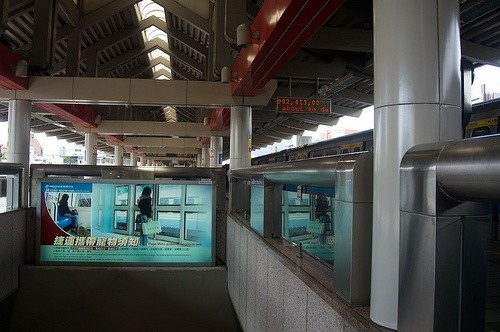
[48,198,56,220]
[315,189,331,248]
[137,187,153,247]
[58,193,79,231]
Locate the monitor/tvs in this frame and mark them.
[276,97,332,115]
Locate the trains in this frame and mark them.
[226,98,500,202]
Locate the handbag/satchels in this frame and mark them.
[135,214,142,224]
[306,218,325,235]
[325,216,331,223]
[142,218,162,235]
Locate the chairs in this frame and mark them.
[56,207,73,232]
